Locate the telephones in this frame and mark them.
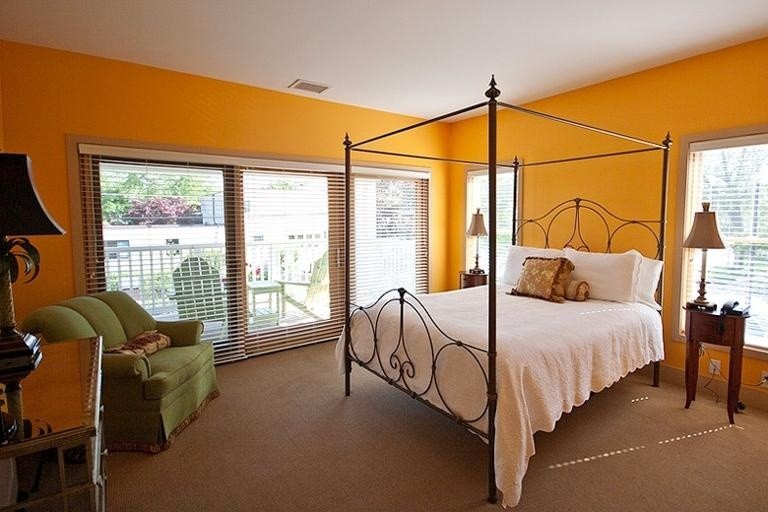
[721,300,751,315]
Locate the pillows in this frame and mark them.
[493,246,667,311]
[102,327,171,357]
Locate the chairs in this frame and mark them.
[19,289,219,455]
[169,257,228,339]
[275,251,329,321]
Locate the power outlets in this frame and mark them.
[709,358,721,375]
[760,371,768,387]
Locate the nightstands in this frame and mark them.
[683,303,751,423]
[459,273,488,289]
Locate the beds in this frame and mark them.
[343,72,674,509]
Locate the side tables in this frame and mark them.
[242,280,283,330]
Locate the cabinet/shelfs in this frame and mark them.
[1,423,108,512]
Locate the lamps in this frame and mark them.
[466,208,488,275]
[682,201,725,312]
[0,153,68,362]
